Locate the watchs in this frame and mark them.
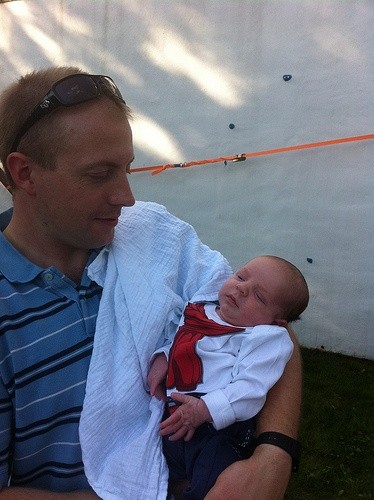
[249,431,303,474]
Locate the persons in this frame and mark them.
[0,66,304,500]
[146,254,310,500]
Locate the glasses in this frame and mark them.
[10,73,127,152]
